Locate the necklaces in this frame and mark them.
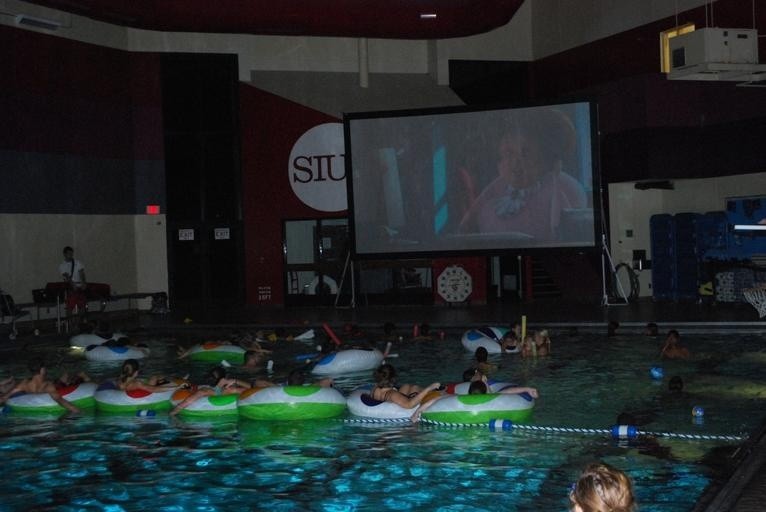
[488,170,559,217]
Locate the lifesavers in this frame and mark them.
[68,332,150,362]
[308,276,338,294]
[4,383,99,415]
[189,342,245,365]
[169,383,239,419]
[420,388,536,424]
[94,381,182,414]
[462,326,519,354]
[238,384,346,423]
[346,384,449,419]
[298,347,384,375]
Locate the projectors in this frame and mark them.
[668,27,759,71]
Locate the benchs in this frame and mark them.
[15,289,167,319]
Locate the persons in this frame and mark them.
[503,324,550,358]
[175,342,192,360]
[457,110,590,238]
[118,361,191,394]
[0,361,90,414]
[373,364,539,409]
[166,367,336,418]
[608,321,691,360]
[60,244,90,326]
[242,328,297,372]
[568,458,637,511]
